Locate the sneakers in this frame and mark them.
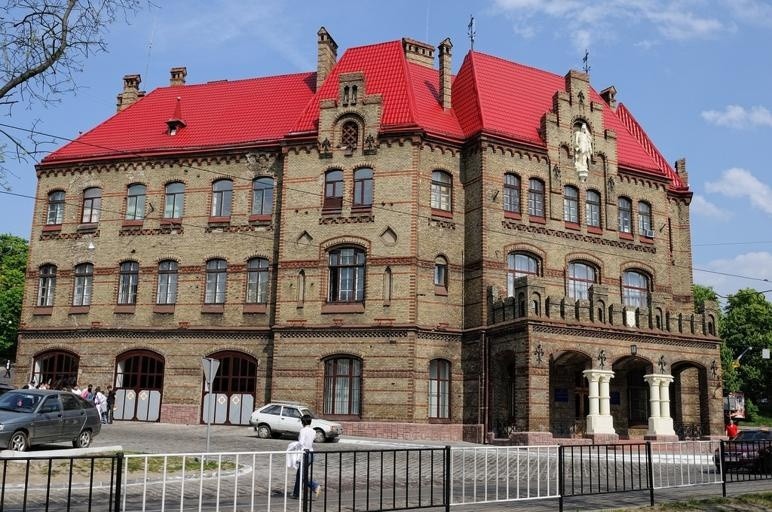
[290,493,298,500]
[316,484,321,497]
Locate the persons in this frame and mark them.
[14,379,117,425]
[725,420,738,442]
[288,415,322,501]
[574,121,593,169]
[3,359,12,379]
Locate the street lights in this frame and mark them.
[735,346,752,362]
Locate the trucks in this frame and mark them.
[722,388,746,422]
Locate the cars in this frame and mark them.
[0,388,102,450]
[1,382,17,396]
[710,426,771,474]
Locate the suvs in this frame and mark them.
[249,400,344,443]
